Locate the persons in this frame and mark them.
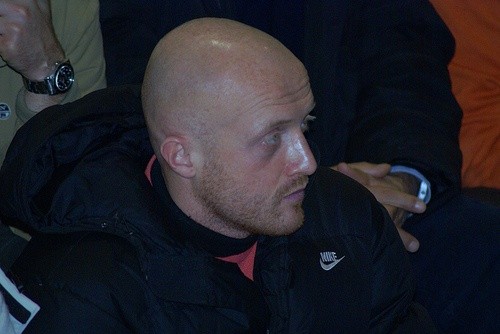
[0,0,108,168]
[0,18,436,334]
[99,0,500,334]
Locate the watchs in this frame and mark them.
[22,59,76,95]
[390,165,432,217]
